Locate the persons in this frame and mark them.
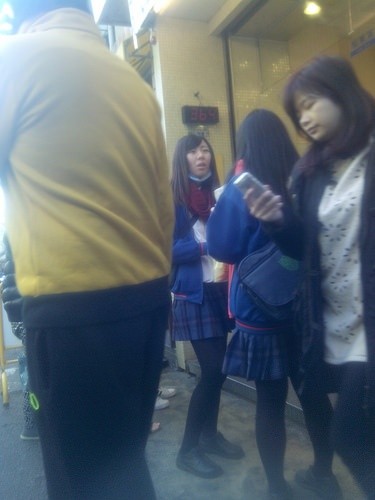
[0,0,176,500]
[150,388,175,432]
[206,110,343,500]
[170,135,245,478]
[0,239,39,440]
[244,59,375,500]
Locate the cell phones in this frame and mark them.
[232,172,274,210]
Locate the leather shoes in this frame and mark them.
[176,448,223,479]
[294,468,341,498]
[197,430,244,459]
[268,482,293,500]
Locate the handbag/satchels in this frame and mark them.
[238,239,306,320]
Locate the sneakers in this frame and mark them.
[153,398,169,410]
[159,388,176,398]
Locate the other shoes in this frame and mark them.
[21,429,40,440]
[149,422,160,432]
